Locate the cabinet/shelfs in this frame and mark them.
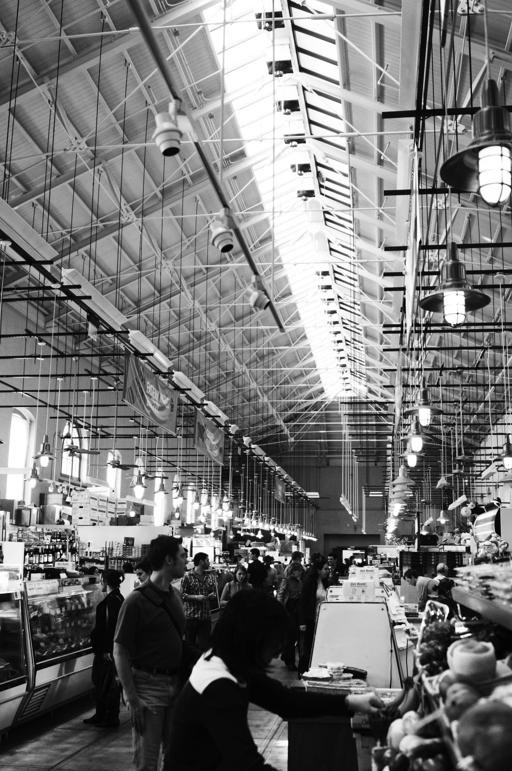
[13,576,108,731]
[370,574,511,771]
[0,590,29,738]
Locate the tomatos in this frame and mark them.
[456,701,511,770]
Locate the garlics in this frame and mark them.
[387,710,426,759]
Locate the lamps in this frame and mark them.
[0,2,512,547]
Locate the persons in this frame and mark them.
[403,569,439,614]
[158,587,385,771]
[132,562,151,590]
[220,547,357,671]
[82,570,124,729]
[123,561,133,573]
[89,566,98,575]
[178,552,215,652]
[112,535,188,771]
[433,562,449,582]
